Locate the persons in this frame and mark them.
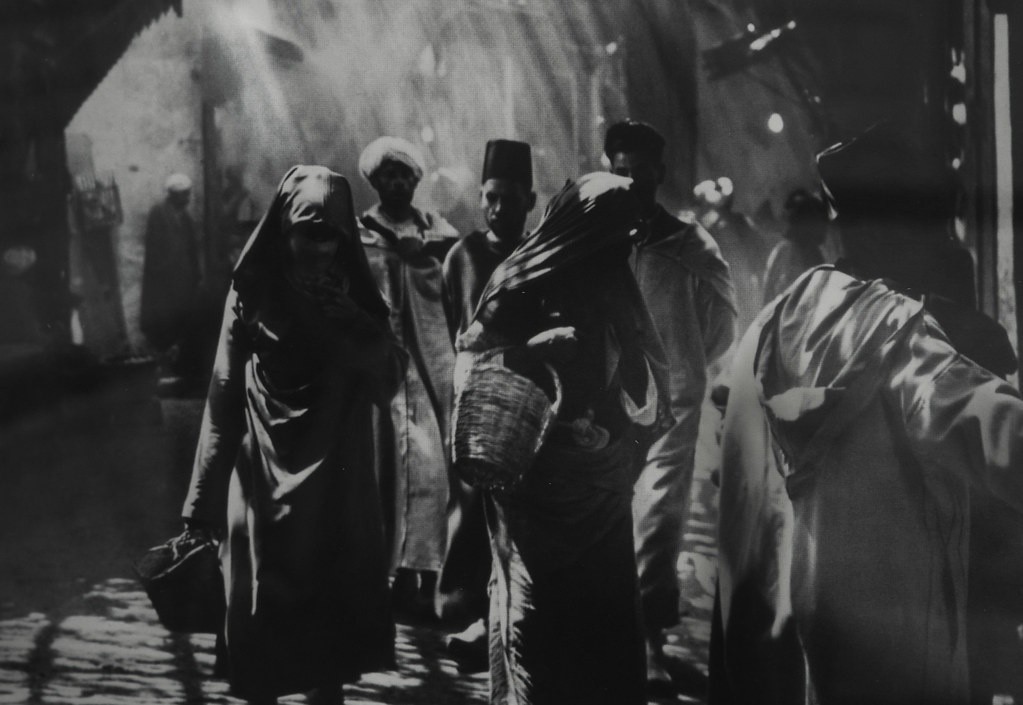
[184,122,1023,705]
[141,174,202,352]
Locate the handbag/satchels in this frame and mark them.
[136,522,226,636]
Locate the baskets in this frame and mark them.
[452,345,562,493]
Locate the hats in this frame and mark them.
[816,119,928,222]
[357,136,424,181]
[482,140,533,187]
[604,120,667,166]
